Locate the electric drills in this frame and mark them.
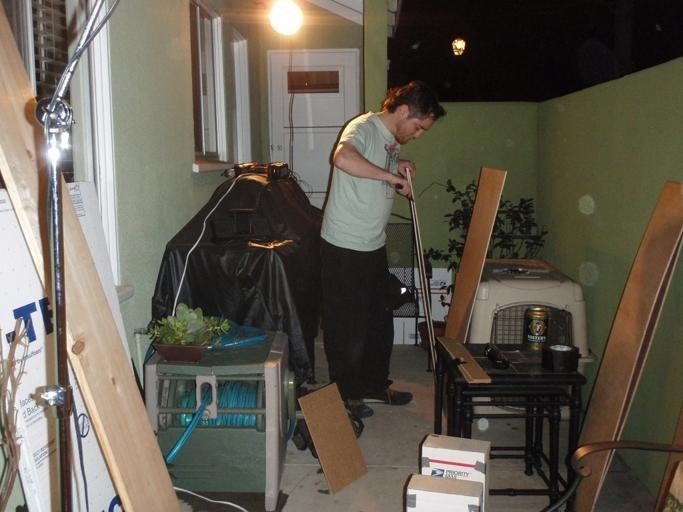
[221,161,288,178]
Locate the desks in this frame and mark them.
[435,342,586,512]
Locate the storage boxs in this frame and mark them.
[421,433,491,512]
[406,474,483,512]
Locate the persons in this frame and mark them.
[319,80,447,418]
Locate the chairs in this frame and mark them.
[571,404,683,512]
[382,212,421,345]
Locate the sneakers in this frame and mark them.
[344,386,414,419]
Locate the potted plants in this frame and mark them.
[145,302,231,364]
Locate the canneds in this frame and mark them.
[523,306,549,352]
